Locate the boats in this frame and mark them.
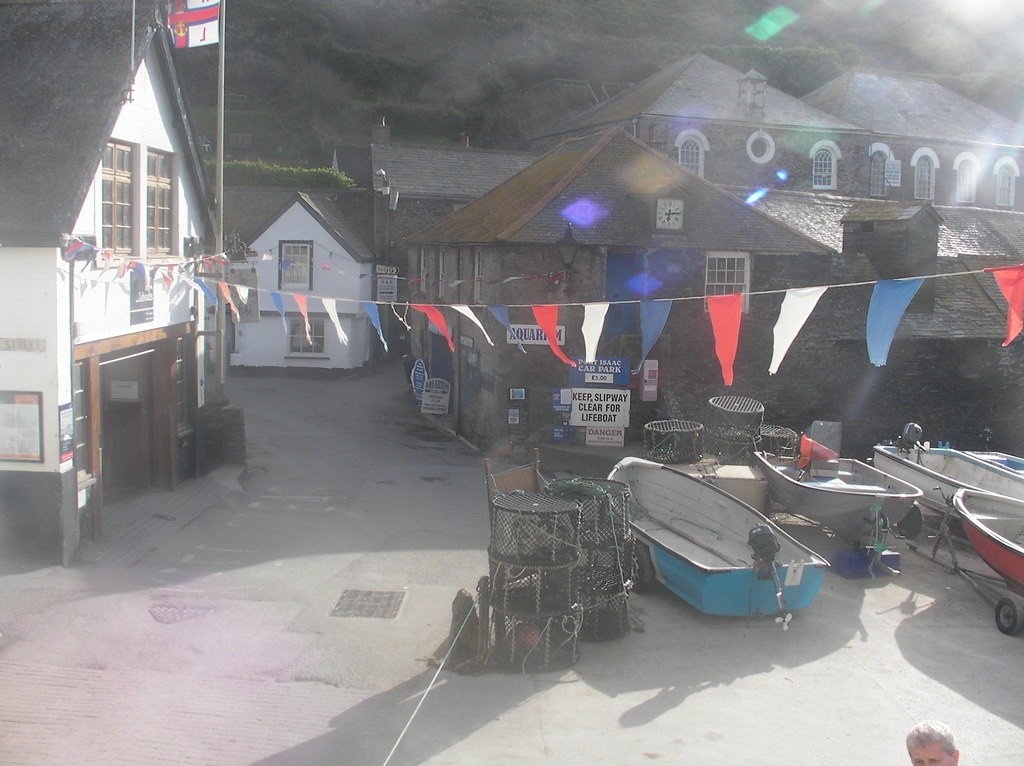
[964,451,1024,478]
[605,456,831,617]
[752,450,924,543]
[872,422,1024,523]
[952,487,1024,594]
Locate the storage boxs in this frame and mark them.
[829,547,900,580]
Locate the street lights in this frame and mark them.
[374,168,399,265]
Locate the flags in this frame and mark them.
[167,0,219,48]
